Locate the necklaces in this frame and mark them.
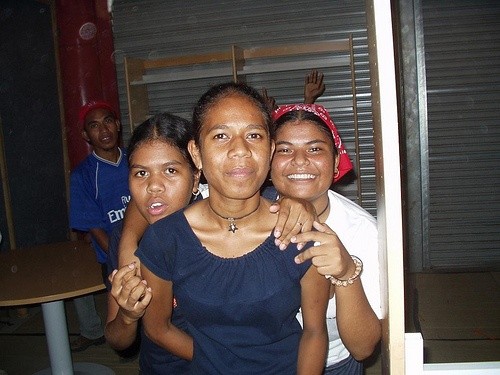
[207,195,262,233]
[316,196,330,217]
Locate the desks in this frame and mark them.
[0,240,116,375]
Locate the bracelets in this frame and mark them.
[324,256,364,287]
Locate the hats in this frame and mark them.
[79,100,119,131]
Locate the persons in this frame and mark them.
[66,70,384,375]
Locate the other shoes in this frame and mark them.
[69,335,106,352]
